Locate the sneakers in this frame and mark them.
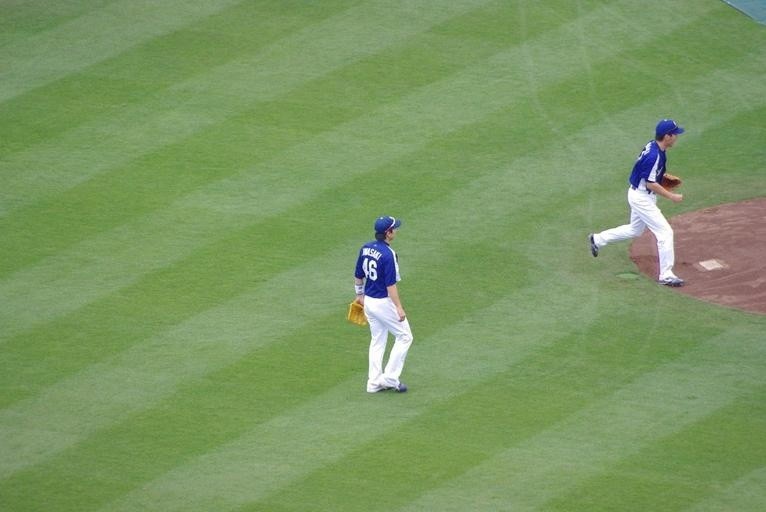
[395,383,407,393]
[590,233,599,257]
[659,277,686,287]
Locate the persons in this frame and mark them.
[354,215,413,394]
[589,119,685,286]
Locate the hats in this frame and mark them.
[655,119,685,136]
[374,216,401,232]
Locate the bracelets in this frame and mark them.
[355,282,364,295]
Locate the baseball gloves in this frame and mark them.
[661,173,681,188]
[347,299,366,326]
